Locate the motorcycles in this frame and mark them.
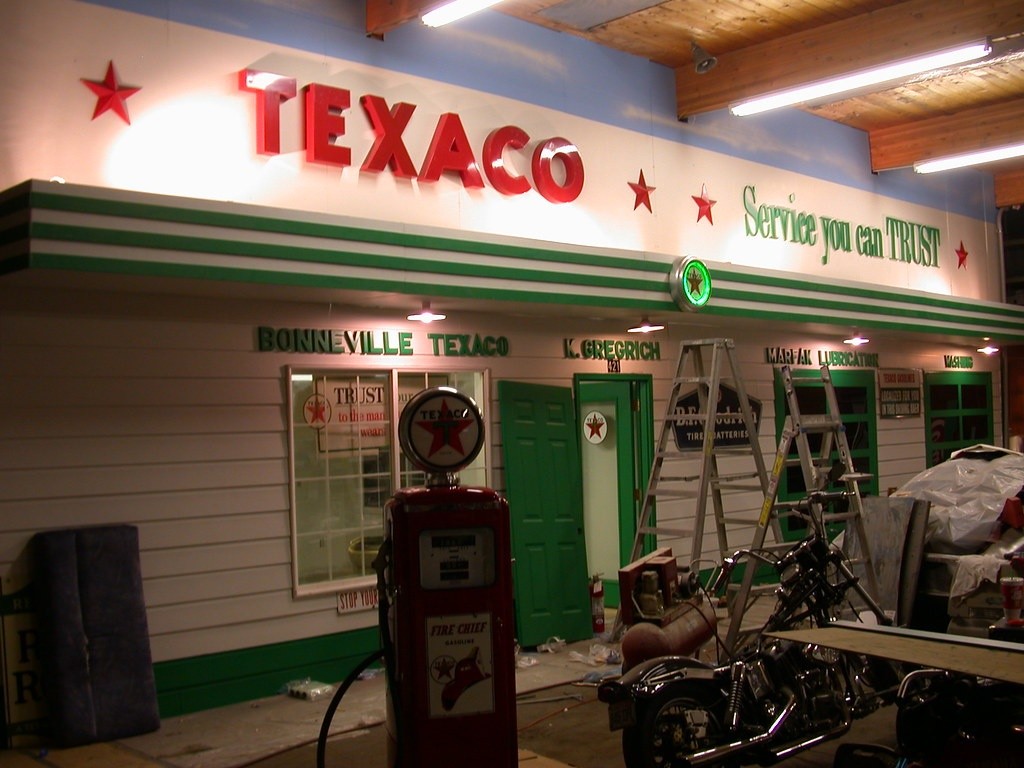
[831,619,1024,767]
[597,490,925,768]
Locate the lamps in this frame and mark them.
[626,318,665,333]
[912,141,1024,175]
[728,36,994,117]
[976,346,999,355]
[844,332,871,345]
[406,301,447,324]
[417,0,504,28]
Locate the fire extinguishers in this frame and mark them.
[588,571,606,633]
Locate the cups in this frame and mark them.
[1001,577,1024,622]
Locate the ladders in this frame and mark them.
[610,336,804,644]
[717,364,887,666]
